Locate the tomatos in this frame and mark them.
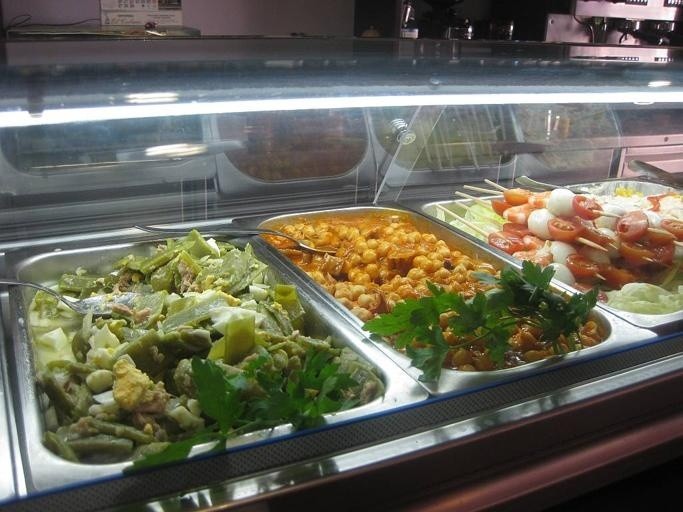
[491,187,683,302]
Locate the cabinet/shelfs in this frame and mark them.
[0,33,682,512]
